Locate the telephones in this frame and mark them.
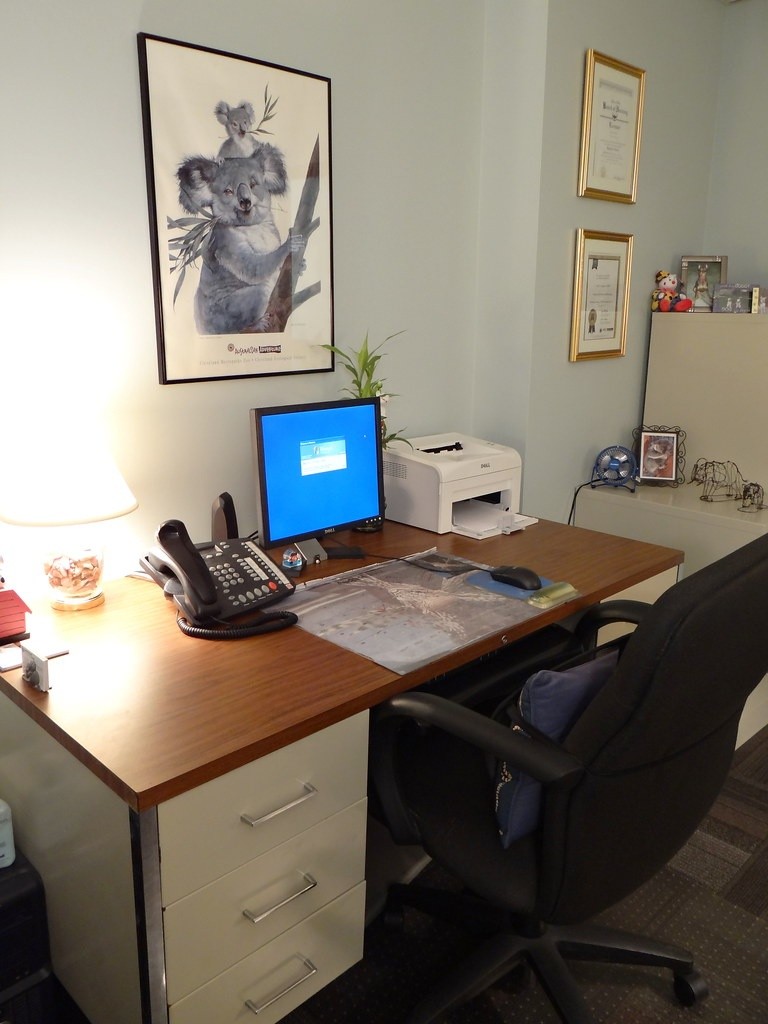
[138,520,297,627]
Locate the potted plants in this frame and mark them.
[317,328,414,533]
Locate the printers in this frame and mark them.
[382,431,539,540]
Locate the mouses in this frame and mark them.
[491,565,542,590]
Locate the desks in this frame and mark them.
[0,514,684,1024]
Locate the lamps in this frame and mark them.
[1,434,141,613]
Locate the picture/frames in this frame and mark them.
[576,48,647,204]
[568,227,634,363]
[631,424,686,488]
[135,31,337,386]
[680,255,728,312]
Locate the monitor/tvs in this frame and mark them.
[250,395,385,577]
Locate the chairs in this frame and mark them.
[369,533,768,1024]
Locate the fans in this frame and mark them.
[590,445,638,493]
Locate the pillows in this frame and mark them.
[493,649,621,850]
